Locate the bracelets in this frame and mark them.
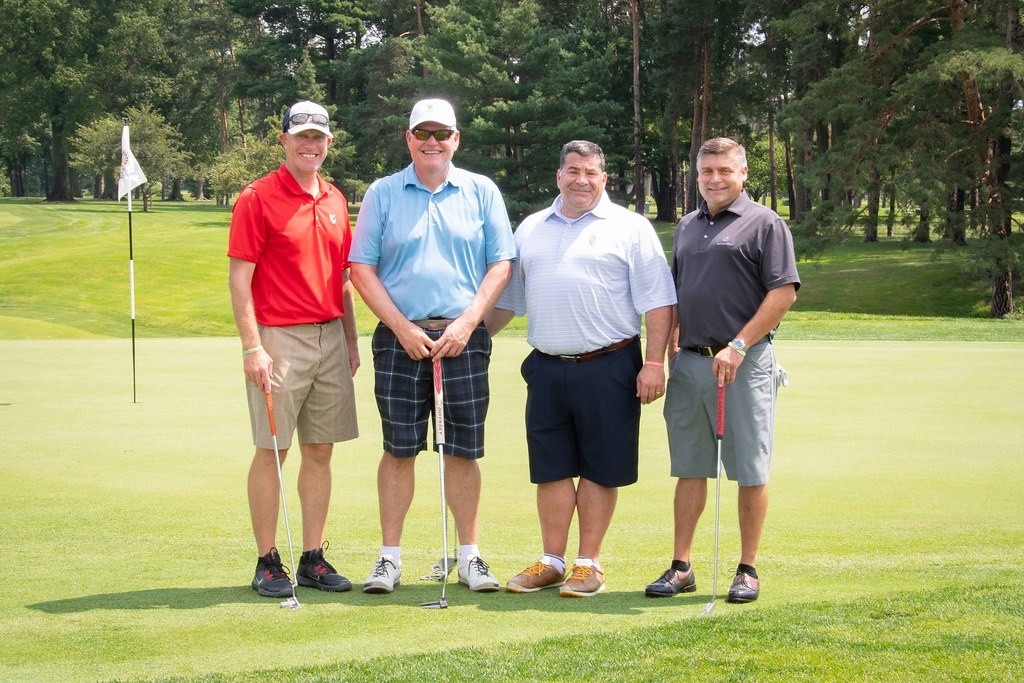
[728,342,746,356]
[645,361,664,366]
[242,345,263,355]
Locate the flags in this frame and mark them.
[119,129,147,201]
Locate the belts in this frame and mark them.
[559,332,637,362]
[684,333,774,358]
[412,318,454,330]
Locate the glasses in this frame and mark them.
[283,113,328,133]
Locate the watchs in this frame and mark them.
[731,339,748,353]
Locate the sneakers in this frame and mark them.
[458,554,499,591]
[296,541,352,592]
[506,561,567,592]
[363,554,402,592]
[252,547,297,597]
[560,559,605,598]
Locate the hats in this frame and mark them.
[412,128,454,141]
[282,100,333,139]
[409,99,456,129]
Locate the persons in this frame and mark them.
[484,140,679,597]
[347,98,517,590]
[645,139,801,603]
[228,100,360,597]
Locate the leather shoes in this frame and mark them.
[644,566,697,596]
[728,568,759,602]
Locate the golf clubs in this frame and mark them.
[420,354,454,609]
[263,382,301,610]
[701,373,726,617]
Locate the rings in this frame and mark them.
[726,370,730,371]
[656,392,661,394]
